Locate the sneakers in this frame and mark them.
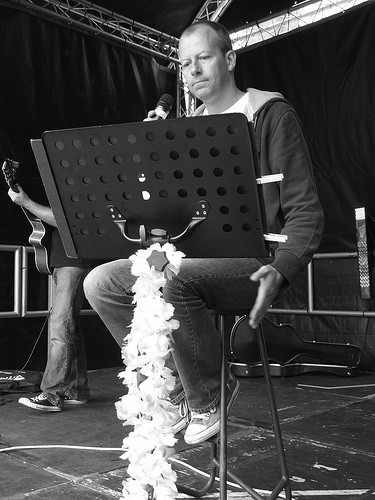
[185,370,241,446]
[18,394,62,412]
[170,412,191,434]
[63,395,87,405]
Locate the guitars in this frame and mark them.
[1,156,54,275]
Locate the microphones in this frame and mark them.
[154,94,174,120]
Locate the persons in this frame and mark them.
[81,20,323,446]
[6,182,91,413]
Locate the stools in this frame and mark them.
[176,309,293,500]
